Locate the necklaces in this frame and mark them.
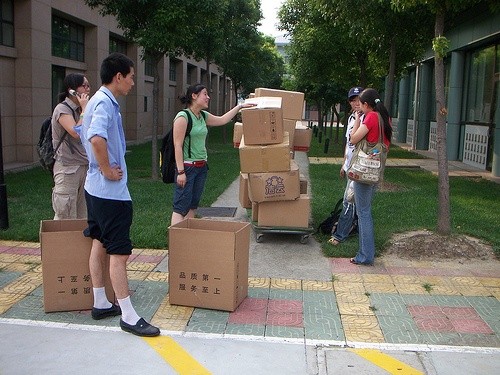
[189,107,200,120]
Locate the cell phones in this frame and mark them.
[69,90,81,98]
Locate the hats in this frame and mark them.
[348,87,364,98]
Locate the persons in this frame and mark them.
[79,53,160,337]
[350,87,393,267]
[51,72,90,220]
[171,84,257,225]
[329,88,364,246]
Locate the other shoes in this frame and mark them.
[328,238,340,245]
[350,257,374,265]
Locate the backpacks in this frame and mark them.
[159,110,206,184]
[37,102,76,175]
[312,197,358,236]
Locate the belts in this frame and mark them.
[184,160,207,164]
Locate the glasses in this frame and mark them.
[81,84,90,88]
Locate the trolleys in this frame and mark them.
[245,207,315,244]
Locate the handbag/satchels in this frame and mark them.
[346,112,387,185]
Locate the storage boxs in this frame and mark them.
[168,87,312,310]
[39,218,115,314]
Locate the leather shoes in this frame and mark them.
[120,317,160,337]
[91,301,122,320]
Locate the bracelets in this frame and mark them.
[80,113,84,117]
[177,170,184,175]
